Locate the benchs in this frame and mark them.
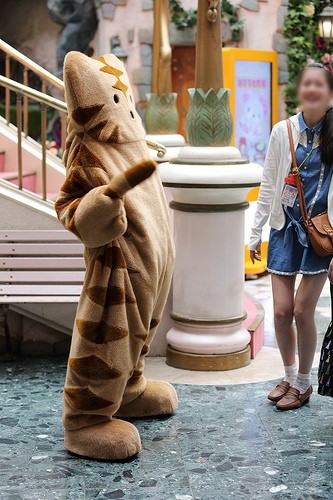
[0,230,86,356]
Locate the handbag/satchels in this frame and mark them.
[317,320,333,398]
[306,211,333,258]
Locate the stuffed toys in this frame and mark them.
[55,50,178,460]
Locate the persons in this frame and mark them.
[248,62,333,409]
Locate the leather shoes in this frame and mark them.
[275,384,313,410]
[268,380,292,402]
